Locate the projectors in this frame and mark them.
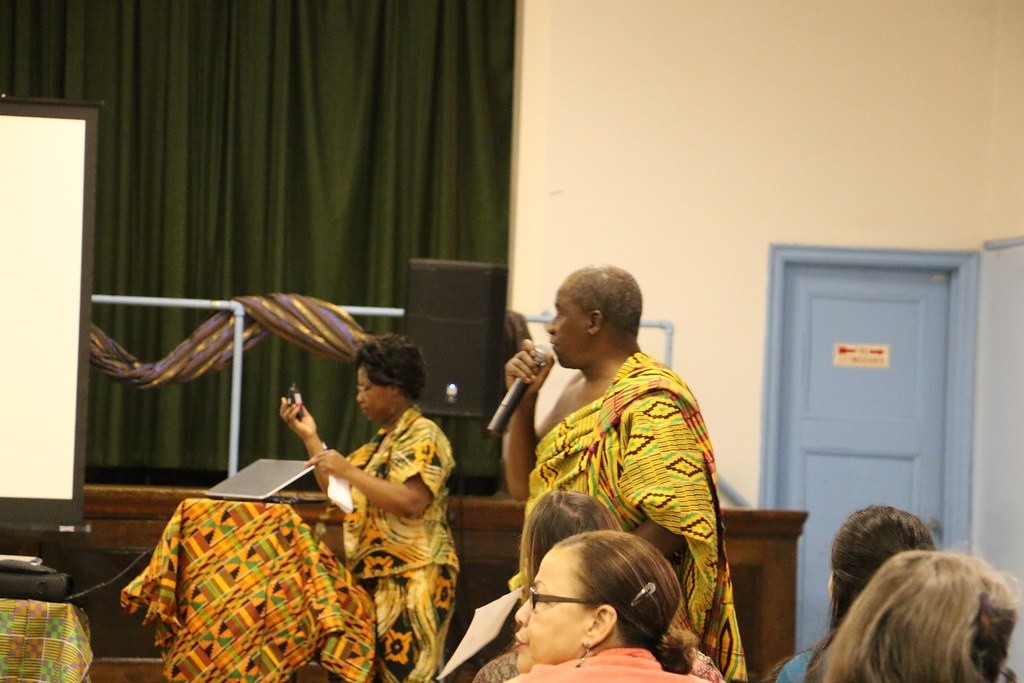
[1,570,97,604]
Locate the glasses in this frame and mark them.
[529,587,600,610]
[356,382,374,392]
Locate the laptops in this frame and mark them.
[203,457,328,506]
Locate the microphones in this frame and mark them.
[486,343,551,435]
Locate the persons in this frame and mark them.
[471,488,731,682]
[501,531,716,683]
[771,504,1015,683]
[826,550,1020,683]
[497,264,749,681]
[279,339,462,683]
